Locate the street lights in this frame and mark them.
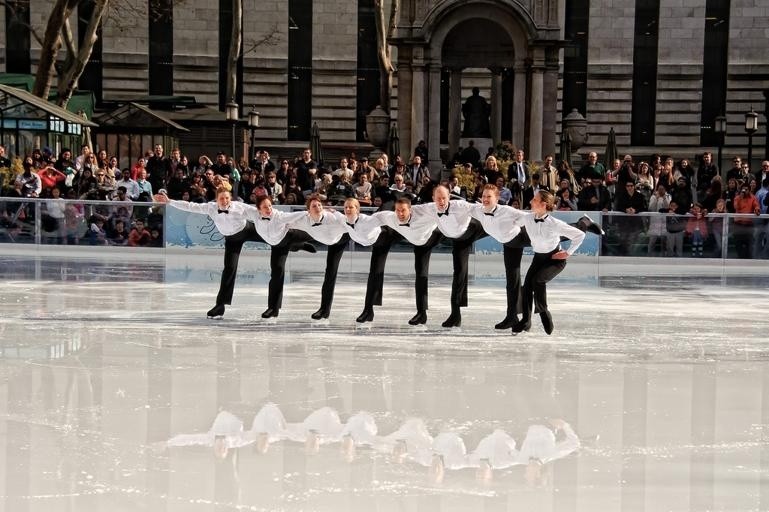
[715,116,726,177]
[226,98,238,160]
[248,105,260,159]
[745,105,758,172]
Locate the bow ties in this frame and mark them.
[437,210,448,217]
[485,212,494,216]
[346,222,354,229]
[218,209,228,214]
[400,223,410,227]
[312,223,321,227]
[262,217,270,220]
[534,218,544,223]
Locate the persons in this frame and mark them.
[327,198,407,323]
[448,180,606,331]
[229,194,314,317]
[0,143,768,259]
[369,195,444,326]
[510,189,587,337]
[459,87,492,139]
[153,183,319,318]
[411,183,491,329]
[286,194,353,319]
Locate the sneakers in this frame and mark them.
[442,314,461,327]
[512,319,531,332]
[261,308,278,318]
[541,313,553,334]
[208,305,224,316]
[409,313,427,325]
[357,308,373,322]
[304,242,316,253]
[312,308,329,319]
[495,317,519,329]
[580,217,602,235]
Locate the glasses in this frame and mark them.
[283,162,288,165]
[268,176,273,179]
[97,174,103,176]
[627,184,633,187]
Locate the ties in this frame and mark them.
[519,165,523,177]
